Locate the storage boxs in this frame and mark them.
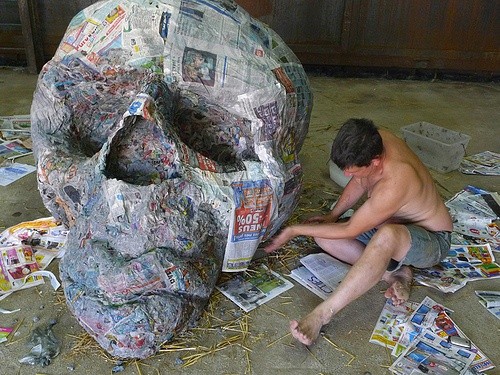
[328,141,352,190]
[399,121,472,174]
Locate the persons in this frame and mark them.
[265,117,451,346]
